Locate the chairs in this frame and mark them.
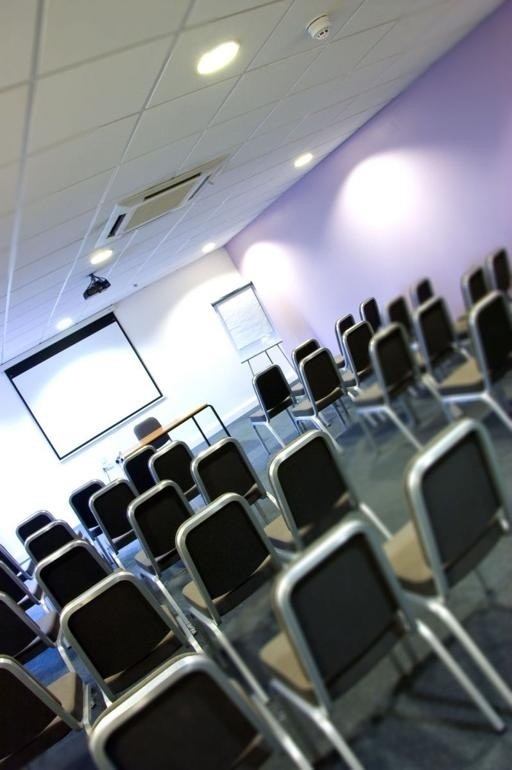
[245,237,512,430]
[3,403,512,769]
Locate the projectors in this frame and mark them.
[82,279,111,300]
